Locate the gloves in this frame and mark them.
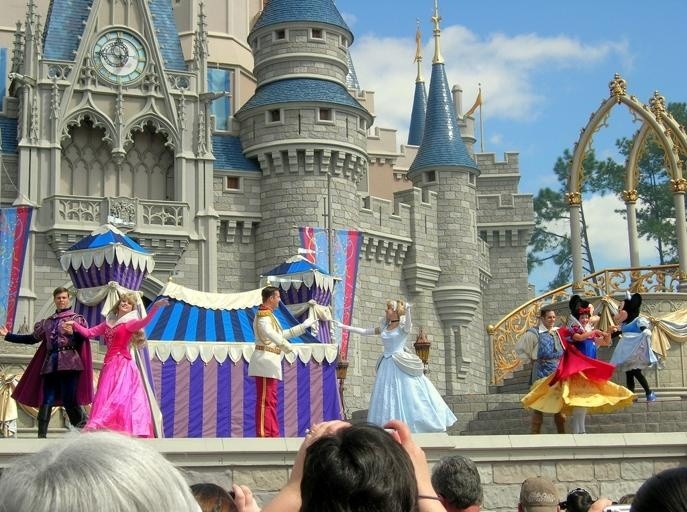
[293,349,297,359]
[403,303,412,333]
[332,320,375,336]
[303,317,319,329]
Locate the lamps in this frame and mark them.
[415,328,432,371]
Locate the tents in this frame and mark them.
[145,276,345,438]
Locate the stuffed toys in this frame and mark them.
[611,293,656,403]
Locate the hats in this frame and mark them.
[520,476,560,506]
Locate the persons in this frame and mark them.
[66,293,170,439]
[333,300,458,433]
[0,287,94,438]
[520,308,567,434]
[247,286,320,438]
[0,419,687,512]
[520,295,636,433]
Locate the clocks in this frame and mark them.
[88,25,151,88]
